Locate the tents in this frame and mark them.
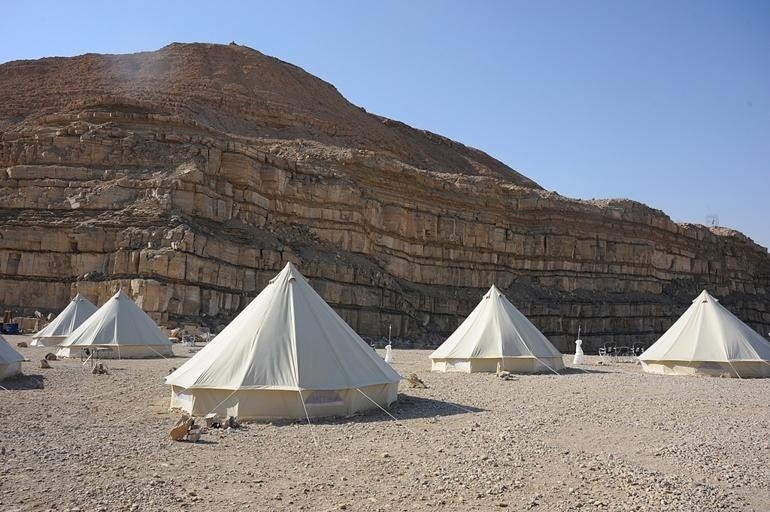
[429,284,565,373]
[59,290,173,358]
[638,289,770,378]
[32,291,100,349]
[164,262,405,417]
[0,335,25,380]
